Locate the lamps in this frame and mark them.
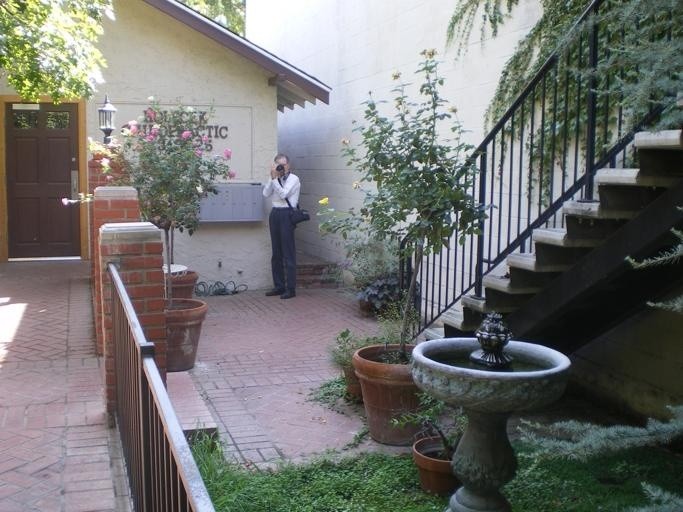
[99,95,118,145]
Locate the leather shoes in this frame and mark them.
[265,291,282,296]
[279,290,296,300]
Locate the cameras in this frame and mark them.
[276,164,285,175]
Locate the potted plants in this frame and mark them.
[122,111,227,381]
[323,330,384,399]
[316,179,404,311]
[388,392,467,493]
[340,47,499,444]
[62,93,238,309]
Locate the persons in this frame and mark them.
[263,154,310,299]
